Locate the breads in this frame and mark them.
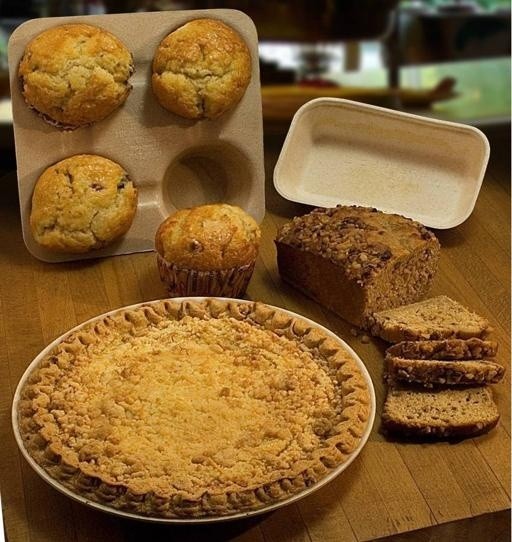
[155,203,261,298]
[151,18,252,121]
[28,154,139,253]
[273,203,505,444]
[18,22,135,131]
